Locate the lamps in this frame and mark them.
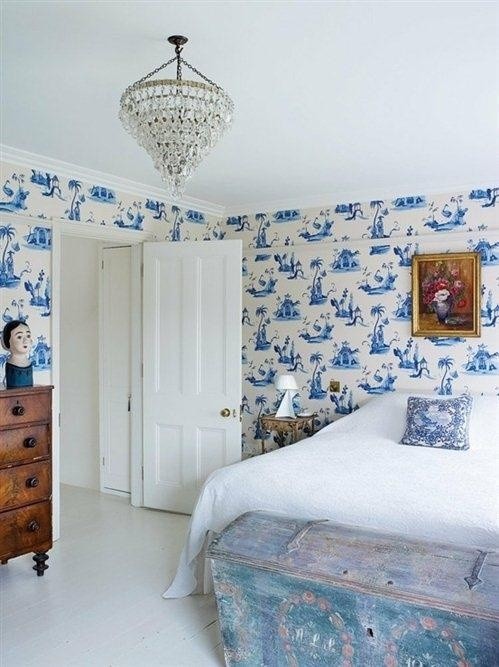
[118,31,232,202]
[274,374,299,419]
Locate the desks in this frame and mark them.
[260,413,314,454]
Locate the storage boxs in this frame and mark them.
[201,509,497,666]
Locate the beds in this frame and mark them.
[165,391,496,604]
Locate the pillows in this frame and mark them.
[398,392,471,448]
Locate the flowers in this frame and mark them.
[422,261,467,309]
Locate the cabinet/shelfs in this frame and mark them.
[0,384,56,575]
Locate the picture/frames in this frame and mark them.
[411,252,481,338]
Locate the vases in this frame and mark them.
[434,301,450,322]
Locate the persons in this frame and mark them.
[0,320,33,389]
